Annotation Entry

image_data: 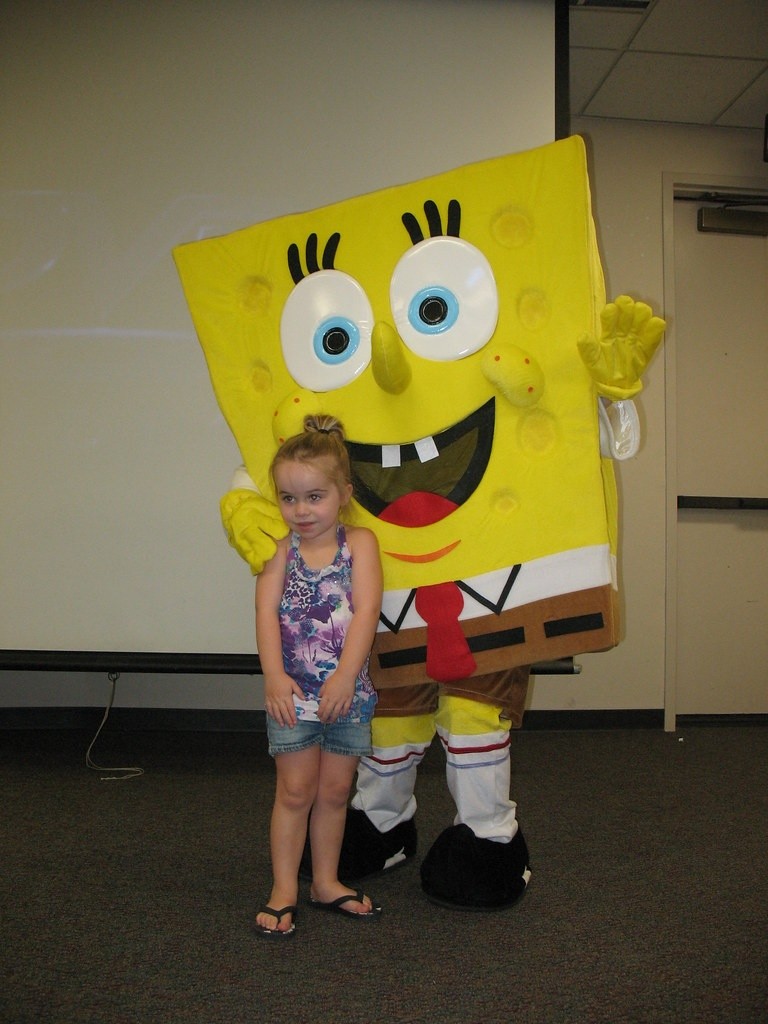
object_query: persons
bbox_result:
[254,415,384,941]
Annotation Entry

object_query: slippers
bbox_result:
[305,887,382,917]
[251,903,299,938]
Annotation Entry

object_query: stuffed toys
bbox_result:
[173,134,667,914]
